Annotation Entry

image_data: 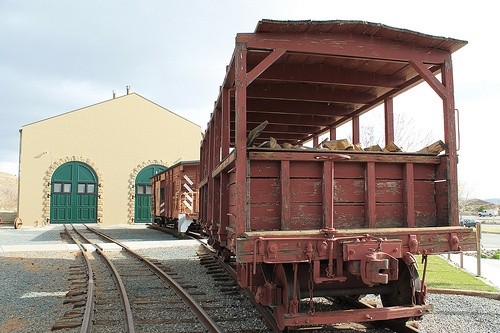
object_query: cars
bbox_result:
[479,210,498,217]
[461,218,480,228]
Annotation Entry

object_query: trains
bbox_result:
[196,17,482,333]
[148,159,202,239]
